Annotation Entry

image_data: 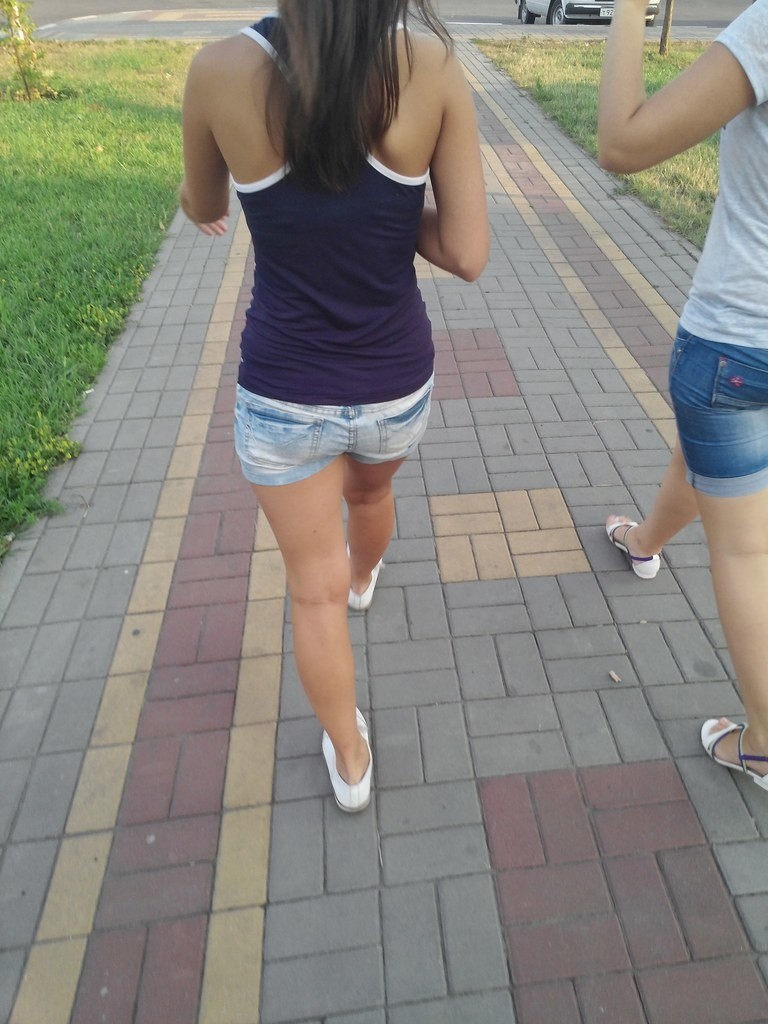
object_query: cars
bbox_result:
[515,0,661,25]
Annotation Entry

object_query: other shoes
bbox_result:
[322,707,373,814]
[347,543,382,610]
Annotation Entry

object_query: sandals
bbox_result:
[701,719,768,790]
[606,518,661,579]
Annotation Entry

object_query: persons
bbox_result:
[593,0,768,786]
[180,0,490,814]
